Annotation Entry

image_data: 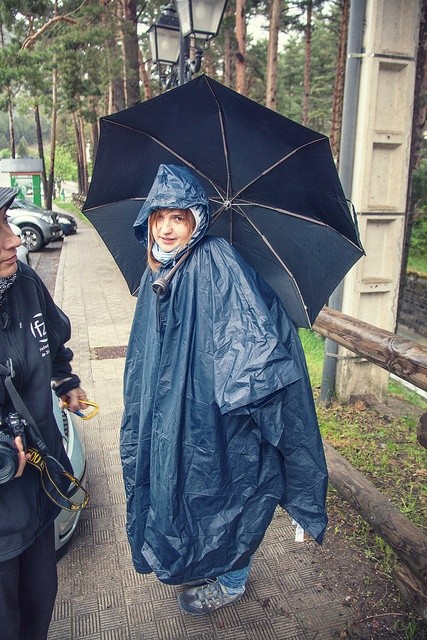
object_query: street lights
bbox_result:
[145,0,230,88]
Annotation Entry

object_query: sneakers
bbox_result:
[178,577,246,616]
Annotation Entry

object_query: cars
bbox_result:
[6,222,31,269]
[49,210,79,236]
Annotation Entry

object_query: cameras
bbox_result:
[0,412,29,485]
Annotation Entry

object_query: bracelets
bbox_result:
[51,374,81,396]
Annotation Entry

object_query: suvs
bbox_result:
[5,191,64,253]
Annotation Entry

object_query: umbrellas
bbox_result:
[82,73,366,331]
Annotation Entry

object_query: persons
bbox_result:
[119,164,328,615]
[0,187,88,640]
